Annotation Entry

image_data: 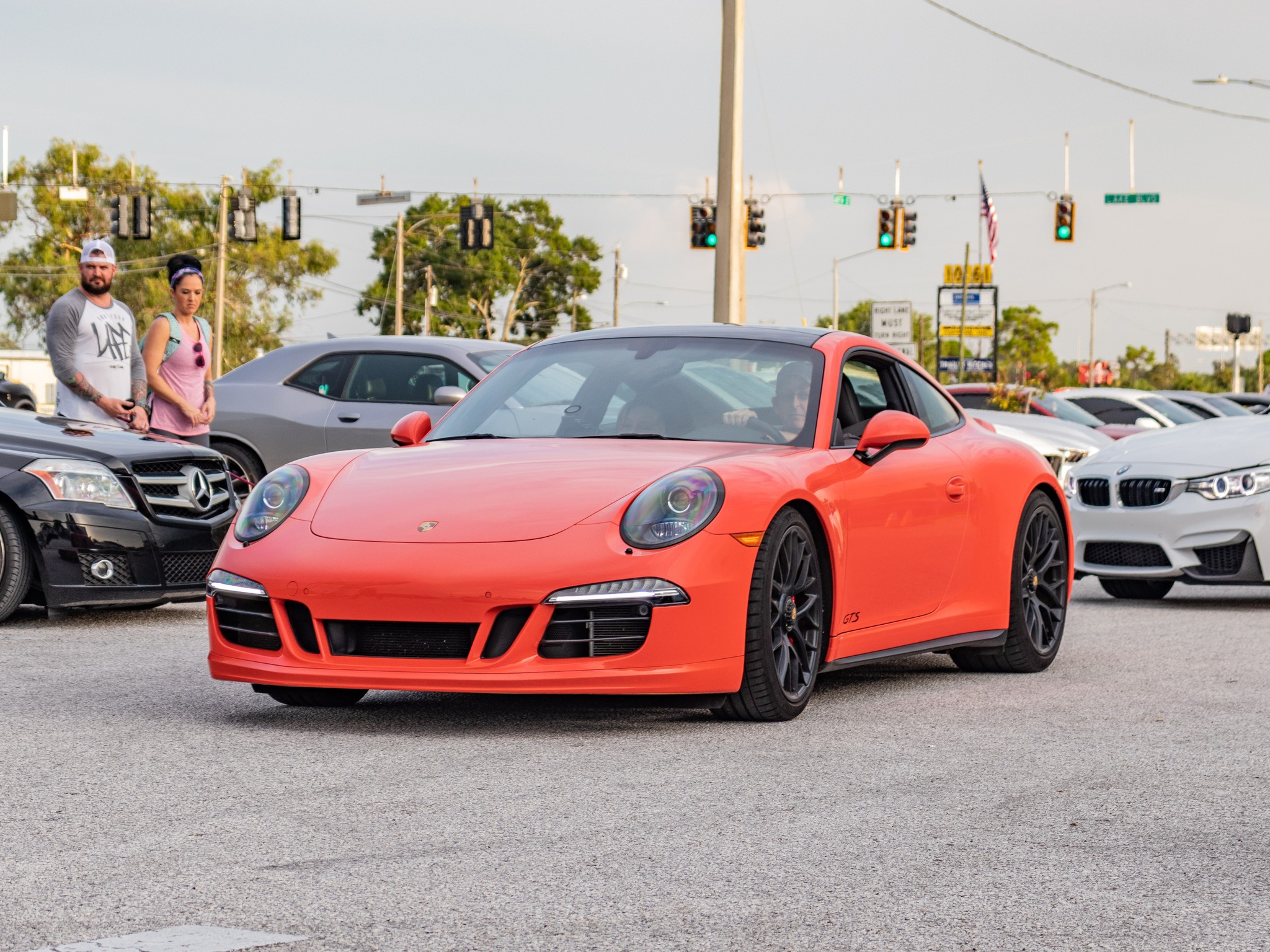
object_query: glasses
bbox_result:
[192,342,205,368]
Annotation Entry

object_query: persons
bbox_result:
[139,252,217,448]
[44,239,153,433]
[720,361,840,452]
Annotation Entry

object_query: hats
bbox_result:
[80,239,116,266]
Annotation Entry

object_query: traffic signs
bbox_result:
[1104,194,1160,203]
[834,193,850,205]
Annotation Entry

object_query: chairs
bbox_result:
[414,374,443,403]
[836,374,863,437]
[358,371,387,402]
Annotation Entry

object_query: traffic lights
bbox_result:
[1228,315,1250,337]
[878,207,897,249]
[691,205,717,249]
[227,196,257,242]
[744,202,766,250]
[1054,201,1075,243]
[110,194,127,239]
[901,208,918,250]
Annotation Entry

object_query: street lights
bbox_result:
[1089,282,1133,386]
[394,210,523,334]
[613,299,669,327]
[832,242,901,331]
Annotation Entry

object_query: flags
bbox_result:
[979,175,1001,266]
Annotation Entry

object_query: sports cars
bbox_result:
[207,326,1076,723]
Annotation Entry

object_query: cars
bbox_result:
[0,407,241,623]
[1061,414,1270,601]
[0,372,37,412]
[208,330,627,503]
[615,356,1270,491]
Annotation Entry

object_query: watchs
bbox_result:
[135,401,151,416]
